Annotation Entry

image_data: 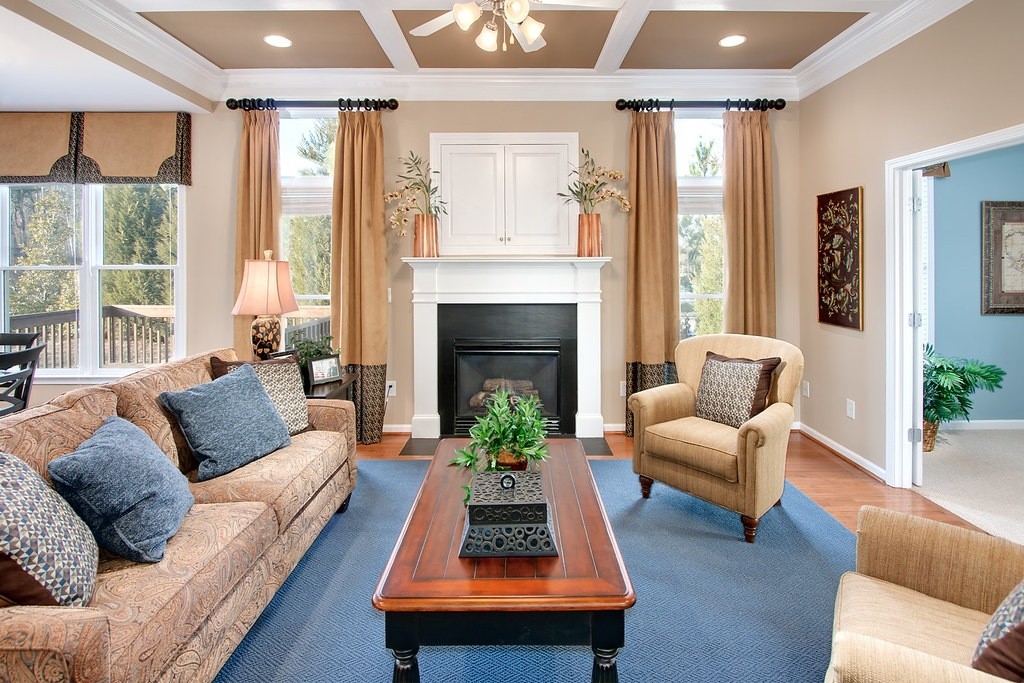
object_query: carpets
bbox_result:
[211,458,857,683]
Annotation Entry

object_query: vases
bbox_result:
[413,211,439,257]
[578,213,603,258]
[251,315,282,361]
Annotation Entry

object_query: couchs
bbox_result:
[628,333,803,542]
[0,347,357,680]
[822,506,1024,683]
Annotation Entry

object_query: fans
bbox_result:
[409,0,627,52]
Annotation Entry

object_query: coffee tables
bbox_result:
[374,437,637,682]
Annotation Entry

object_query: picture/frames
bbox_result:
[980,200,1024,314]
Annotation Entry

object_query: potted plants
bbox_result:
[922,344,1007,452]
[448,385,551,505]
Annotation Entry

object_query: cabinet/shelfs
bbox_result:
[429,132,578,256]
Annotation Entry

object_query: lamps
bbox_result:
[230,249,298,316]
[454,1,545,51]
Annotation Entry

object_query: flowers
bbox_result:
[556,147,632,214]
[382,150,449,236]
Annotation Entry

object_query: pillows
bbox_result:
[0,450,99,610]
[694,351,781,429]
[971,575,1024,683]
[160,364,292,481]
[47,415,195,564]
[211,355,317,438]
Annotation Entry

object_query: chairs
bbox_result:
[0,332,46,417]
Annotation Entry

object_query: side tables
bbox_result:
[303,373,359,401]
